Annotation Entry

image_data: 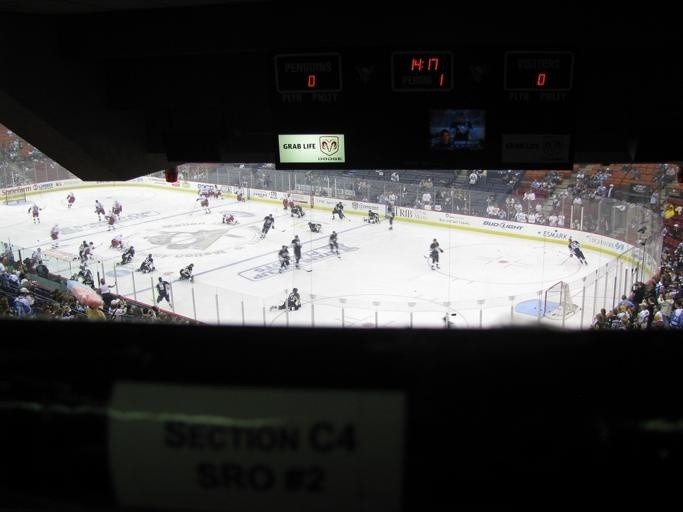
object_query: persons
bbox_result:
[196,193,211,215]
[220,214,237,224]
[0,192,195,327]
[307,222,321,232]
[289,206,304,218]
[590,165,682,329]
[282,193,294,210]
[276,245,289,274]
[566,238,588,267]
[353,162,612,233]
[329,231,341,255]
[290,235,302,267]
[429,238,443,270]
[430,110,480,147]
[269,288,301,312]
[260,214,274,237]
[331,202,343,220]
[0,125,72,191]
[181,161,353,202]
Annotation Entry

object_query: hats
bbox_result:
[19,287,29,293]
[110,298,120,305]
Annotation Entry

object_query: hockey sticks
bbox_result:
[343,216,351,221]
[289,258,312,272]
